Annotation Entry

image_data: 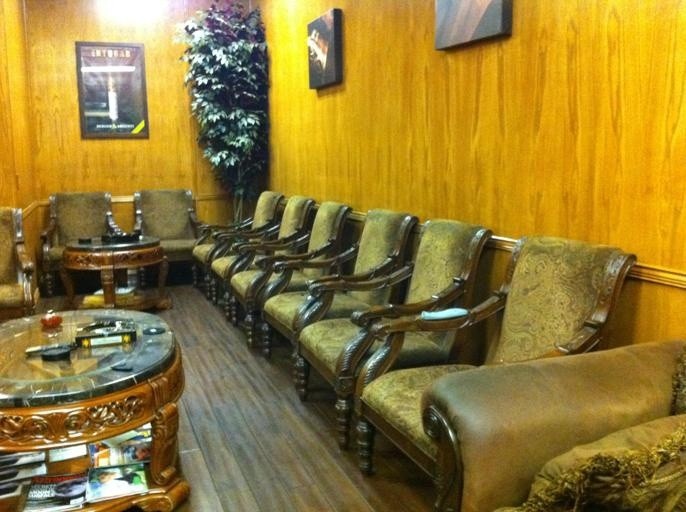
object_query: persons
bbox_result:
[307,18,336,88]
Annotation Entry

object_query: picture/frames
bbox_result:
[73,38,152,143]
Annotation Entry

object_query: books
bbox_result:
[0,421,154,511]
[75,329,138,347]
